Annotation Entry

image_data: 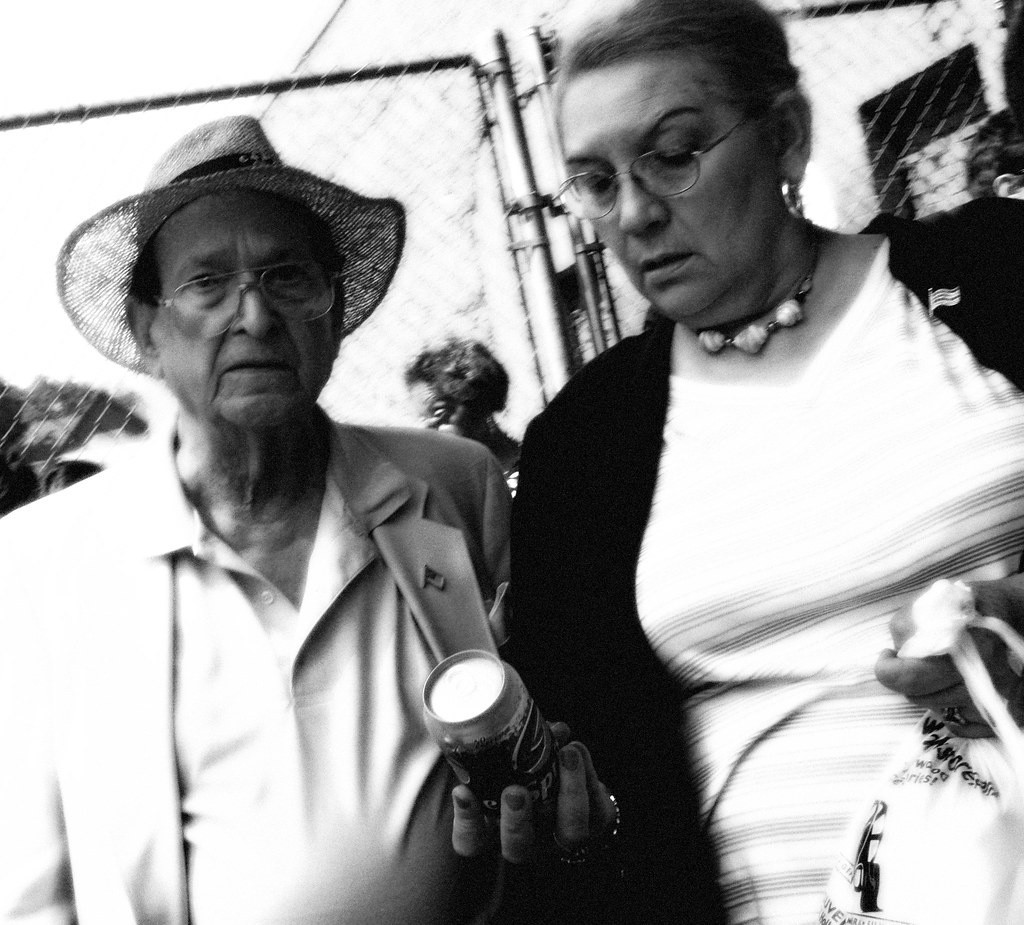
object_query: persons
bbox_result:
[965,106,1023,197]
[0,117,511,925]
[402,335,522,499]
[450,0,1023,925]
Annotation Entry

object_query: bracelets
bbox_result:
[605,795,619,836]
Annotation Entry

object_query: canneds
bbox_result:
[423,648,561,828]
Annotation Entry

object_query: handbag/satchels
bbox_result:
[820,582,1024,925]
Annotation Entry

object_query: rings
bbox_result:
[552,831,585,866]
[942,706,969,728]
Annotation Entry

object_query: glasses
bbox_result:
[551,115,747,220]
[154,261,335,337]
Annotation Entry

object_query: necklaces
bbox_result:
[698,218,819,356]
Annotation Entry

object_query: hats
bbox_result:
[57,115,407,378]
